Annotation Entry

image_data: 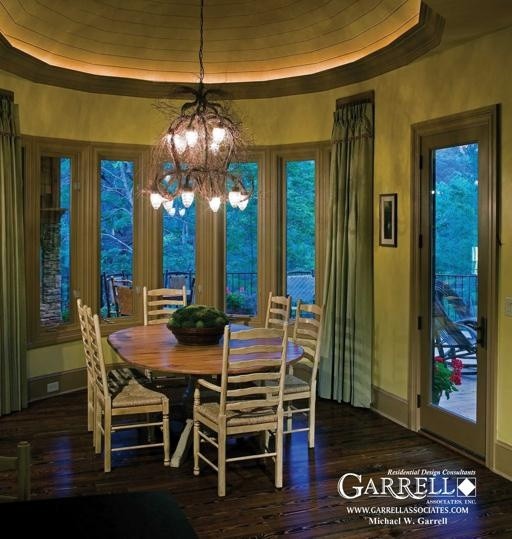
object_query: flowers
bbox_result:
[432,357,464,406]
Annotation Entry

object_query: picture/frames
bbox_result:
[379,193,397,247]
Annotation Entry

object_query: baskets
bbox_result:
[166,323,226,347]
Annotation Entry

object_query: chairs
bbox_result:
[432,279,478,377]
[0,440,33,500]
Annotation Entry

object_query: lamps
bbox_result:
[141,0,254,218]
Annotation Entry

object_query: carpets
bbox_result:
[0,489,198,539]
